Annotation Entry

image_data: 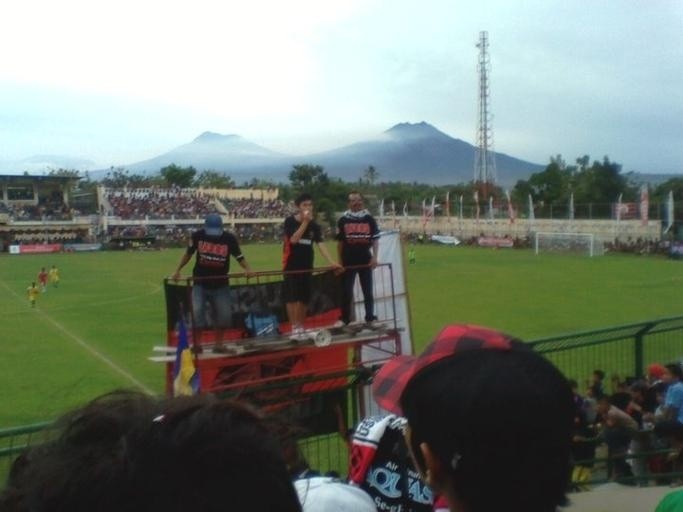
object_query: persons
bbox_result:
[48,265,59,288]
[25,282,38,308]
[566,364,682,489]
[370,323,577,512]
[418,231,533,250]
[0,387,302,512]
[37,267,47,293]
[334,191,379,329]
[240,294,282,338]
[1,192,298,244]
[272,402,452,512]
[279,192,345,335]
[603,235,682,261]
[171,214,252,356]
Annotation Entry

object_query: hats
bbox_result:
[372,325,575,451]
[203,214,224,236]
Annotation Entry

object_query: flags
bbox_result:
[164,265,357,442]
[380,193,515,226]
[616,182,674,234]
[169,317,201,397]
